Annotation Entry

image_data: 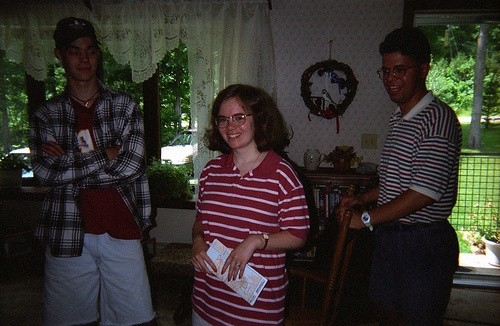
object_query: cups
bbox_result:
[303,149,327,172]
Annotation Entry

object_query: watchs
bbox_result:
[360,210,374,231]
[262,234,269,250]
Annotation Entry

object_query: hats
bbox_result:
[52,17,102,51]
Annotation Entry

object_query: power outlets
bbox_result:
[361,135,379,150]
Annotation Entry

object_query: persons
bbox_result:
[331,25,461,326]
[190,84,310,326]
[29,17,157,326]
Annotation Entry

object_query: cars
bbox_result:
[162,129,198,178]
[0,147,34,178]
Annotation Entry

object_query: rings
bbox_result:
[237,263,241,267]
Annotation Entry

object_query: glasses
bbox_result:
[215,114,254,127]
[376,65,419,80]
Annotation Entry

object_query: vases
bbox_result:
[331,157,352,169]
[482,231,500,265]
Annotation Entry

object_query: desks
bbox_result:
[301,171,382,217]
[0,182,162,256]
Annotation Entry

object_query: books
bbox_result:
[308,186,343,231]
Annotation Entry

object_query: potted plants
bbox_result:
[0,152,30,187]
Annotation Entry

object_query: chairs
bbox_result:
[284,182,361,326]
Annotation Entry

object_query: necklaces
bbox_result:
[249,148,263,168]
[70,89,100,107]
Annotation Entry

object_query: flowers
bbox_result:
[463,203,500,244]
[326,145,357,165]
[301,61,358,121]
